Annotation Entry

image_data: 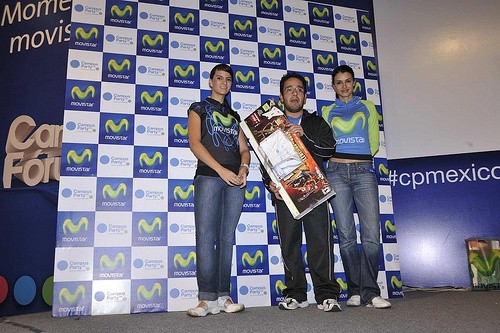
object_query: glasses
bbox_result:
[283,88,304,95]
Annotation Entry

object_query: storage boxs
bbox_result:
[238,97,335,220]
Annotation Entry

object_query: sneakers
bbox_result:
[365,297,392,307]
[346,295,360,305]
[317,299,342,312]
[279,297,309,309]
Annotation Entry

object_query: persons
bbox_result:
[321,65,392,309]
[187,63,250,317]
[259,72,342,312]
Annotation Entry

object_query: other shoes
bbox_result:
[218,296,244,313]
[187,301,220,317]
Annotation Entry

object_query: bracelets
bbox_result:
[240,163,249,169]
